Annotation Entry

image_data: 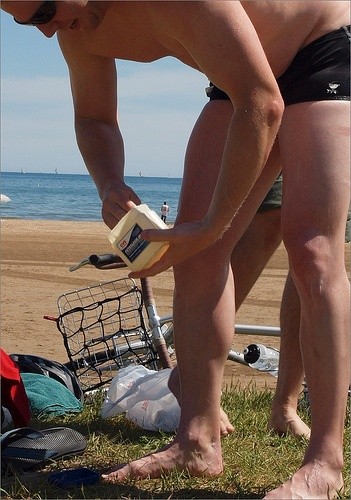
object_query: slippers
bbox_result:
[1,427,87,476]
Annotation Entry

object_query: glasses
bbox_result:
[14,0,56,27]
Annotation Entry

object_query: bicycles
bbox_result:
[55,199,282,397]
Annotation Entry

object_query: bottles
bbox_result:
[243,343,280,373]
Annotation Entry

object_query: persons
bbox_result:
[0,0,351,500]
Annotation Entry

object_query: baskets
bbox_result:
[56,278,158,396]
